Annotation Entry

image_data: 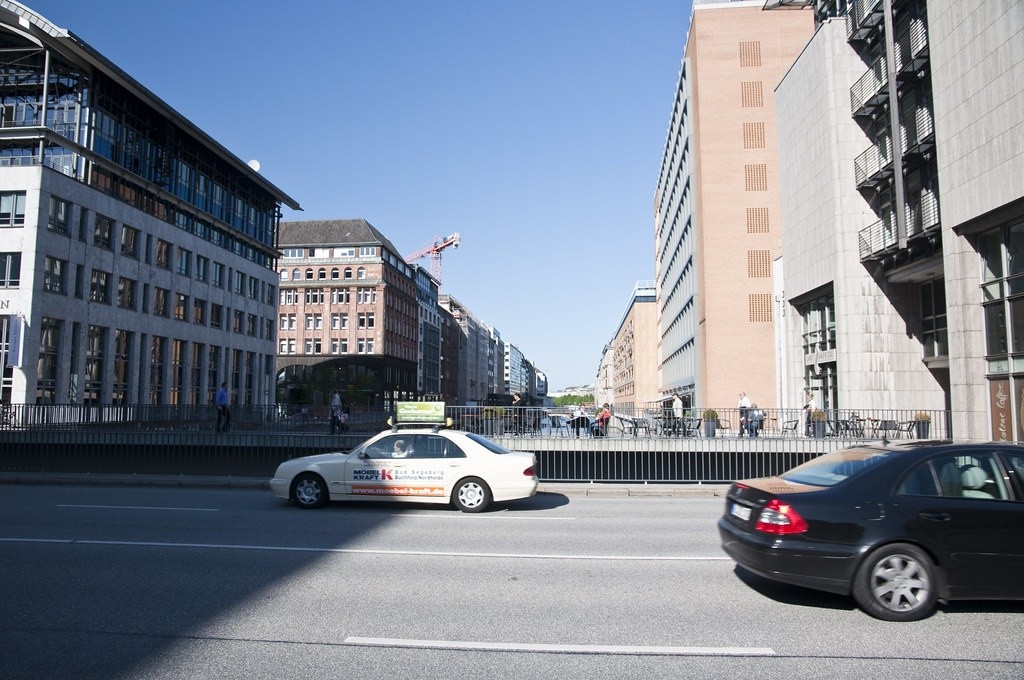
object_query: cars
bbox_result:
[271,418,539,513]
[717,438,1024,622]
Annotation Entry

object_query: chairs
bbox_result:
[654,416,680,437]
[716,419,734,438]
[631,418,651,439]
[760,418,799,438]
[526,417,542,438]
[405,442,426,457]
[547,413,571,438]
[614,415,636,438]
[827,419,866,439]
[959,464,995,499]
[682,418,702,438]
[868,418,916,440]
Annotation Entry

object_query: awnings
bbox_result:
[648,390,689,402]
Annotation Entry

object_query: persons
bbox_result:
[738,391,767,437]
[328,388,350,435]
[512,392,524,435]
[215,382,231,433]
[302,404,308,423]
[668,393,686,436]
[588,403,611,437]
[805,394,816,438]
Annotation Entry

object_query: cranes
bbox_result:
[405,232,461,294]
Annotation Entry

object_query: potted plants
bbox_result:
[914,412,931,439]
[809,407,827,438]
[481,406,506,438]
[702,409,718,437]
